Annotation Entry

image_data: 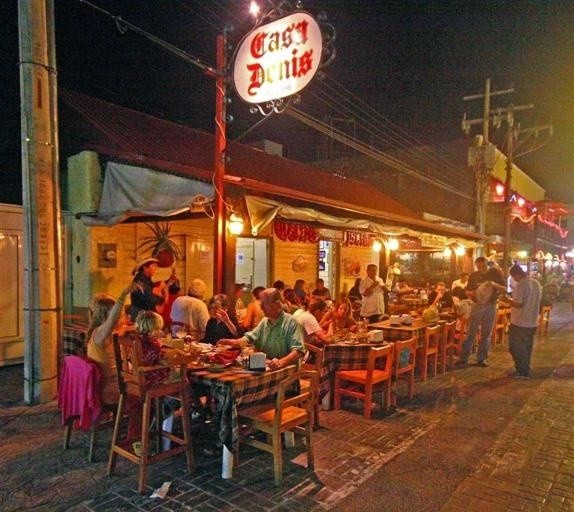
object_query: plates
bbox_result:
[209,365,225,372]
[184,362,212,369]
[194,341,214,352]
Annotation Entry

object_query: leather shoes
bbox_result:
[453,359,467,365]
[478,360,489,367]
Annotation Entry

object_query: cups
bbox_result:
[185,335,191,352]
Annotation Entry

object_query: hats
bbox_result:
[132,254,160,275]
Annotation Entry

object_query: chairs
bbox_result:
[300,299,551,429]
[63,355,115,464]
[233,370,321,486]
[107,333,195,494]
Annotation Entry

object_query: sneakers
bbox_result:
[508,371,529,379]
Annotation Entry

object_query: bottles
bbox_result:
[362,317,368,334]
[358,315,362,336]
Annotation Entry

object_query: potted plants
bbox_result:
[133,222,181,267]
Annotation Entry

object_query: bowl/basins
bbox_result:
[356,334,369,343]
[172,339,184,346]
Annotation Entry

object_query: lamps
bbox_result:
[229,213,243,234]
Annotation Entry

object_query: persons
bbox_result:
[500,265,541,378]
[87,258,305,453]
[507,275,514,296]
[294,264,473,364]
[487,261,497,271]
[456,257,506,367]
